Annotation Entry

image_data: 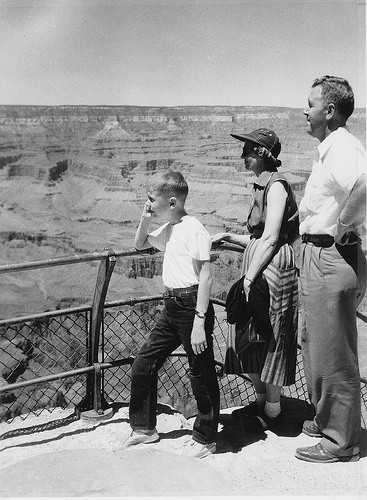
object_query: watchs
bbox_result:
[195,311,206,318]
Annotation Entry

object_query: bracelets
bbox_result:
[245,277,253,282]
[338,218,351,228]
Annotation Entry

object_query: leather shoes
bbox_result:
[294,442,360,464]
[297,419,325,437]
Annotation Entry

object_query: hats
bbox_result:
[229,127,281,159]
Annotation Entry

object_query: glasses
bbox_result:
[242,147,255,156]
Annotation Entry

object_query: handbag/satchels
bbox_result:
[234,316,267,355]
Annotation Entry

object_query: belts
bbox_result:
[164,284,199,298]
[301,233,334,245]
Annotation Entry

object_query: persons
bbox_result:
[118,169,220,458]
[287,75,367,463]
[210,128,300,431]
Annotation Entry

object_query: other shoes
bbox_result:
[181,437,216,460]
[244,411,284,434]
[232,398,265,422]
[112,426,160,452]
